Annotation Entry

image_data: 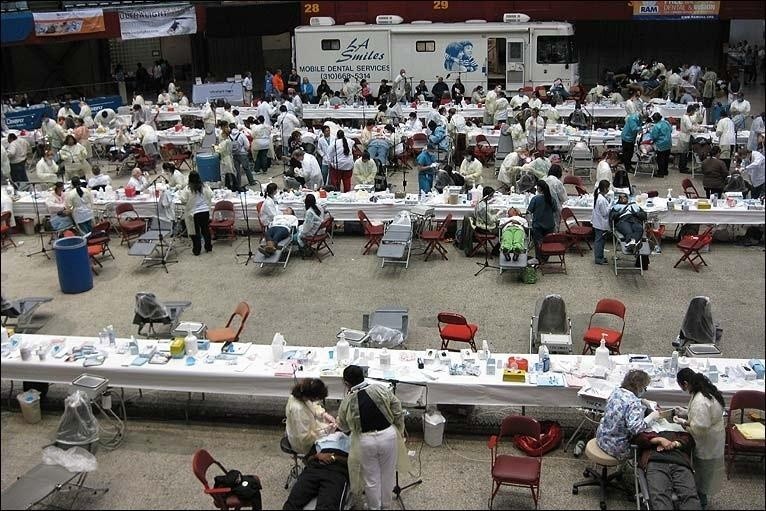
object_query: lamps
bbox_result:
[168,7,180,31]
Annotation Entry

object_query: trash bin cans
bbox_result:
[421,413,446,447]
[16,392,42,424]
[22,219,35,236]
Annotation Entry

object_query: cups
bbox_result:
[329,351,334,359]
[672,125,677,134]
[182,323,198,366]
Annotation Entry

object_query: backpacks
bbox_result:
[229,133,242,156]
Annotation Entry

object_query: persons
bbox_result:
[285,378,337,454]
[596,370,661,501]
[636,404,703,510]
[335,365,404,510]
[672,368,726,505]
[283,427,353,510]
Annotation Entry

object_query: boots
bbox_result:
[257,241,277,257]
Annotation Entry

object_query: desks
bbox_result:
[1,334,766,427]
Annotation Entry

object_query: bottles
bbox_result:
[335,332,350,364]
[533,343,550,374]
[100,185,126,200]
[663,352,720,383]
[420,183,766,214]
[545,123,620,138]
[353,348,397,368]
[212,183,404,202]
[477,341,490,362]
[1,329,45,362]
[6,182,14,197]
[147,181,167,198]
[20,129,26,136]
[573,441,586,457]
[99,326,140,355]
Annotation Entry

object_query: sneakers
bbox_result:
[654,170,669,179]
[502,247,511,261]
[625,167,635,174]
[513,247,520,261]
[680,166,691,175]
[624,239,644,253]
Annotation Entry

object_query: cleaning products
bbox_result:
[184,328,198,354]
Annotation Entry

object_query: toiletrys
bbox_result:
[666,189,673,202]
[466,180,479,202]
[272,333,286,362]
[595,333,610,365]
[336,333,349,360]
[380,346,391,366]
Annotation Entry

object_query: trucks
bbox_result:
[288,13,579,107]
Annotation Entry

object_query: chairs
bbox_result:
[630,444,650,510]
[335,309,409,349]
[608,38,766,87]
[1,82,765,276]
[1,296,54,334]
[581,298,626,355]
[2,459,108,510]
[671,296,723,358]
[437,313,479,349]
[727,390,766,479]
[133,293,192,338]
[529,295,571,355]
[205,301,249,342]
[488,416,541,510]
[192,450,261,511]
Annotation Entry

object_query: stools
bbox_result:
[573,437,633,509]
[280,437,303,489]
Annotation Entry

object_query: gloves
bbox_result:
[430,162,437,170]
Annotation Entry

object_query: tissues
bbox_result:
[438,350,452,365]
[196,340,211,350]
[424,347,435,365]
[737,363,756,380]
[460,349,474,363]
[628,353,652,362]
